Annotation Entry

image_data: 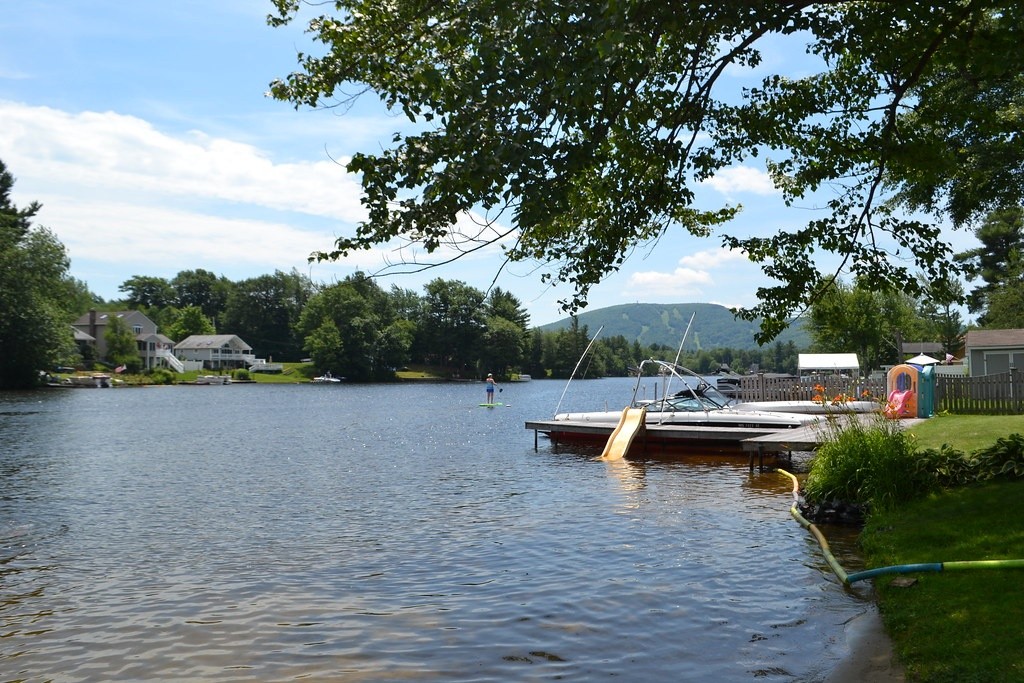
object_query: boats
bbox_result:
[510,374,532,382]
[68,371,113,389]
[538,311,883,448]
[313,372,341,384]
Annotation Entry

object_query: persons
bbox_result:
[487,373,497,403]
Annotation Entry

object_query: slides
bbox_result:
[883,389,912,417]
[599,407,646,460]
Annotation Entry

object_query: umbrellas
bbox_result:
[905,353,940,366]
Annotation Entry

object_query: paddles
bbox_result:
[495,385,504,393]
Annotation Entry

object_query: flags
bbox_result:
[115,364,126,372]
[946,353,954,363]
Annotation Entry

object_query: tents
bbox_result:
[796,352,860,382]
